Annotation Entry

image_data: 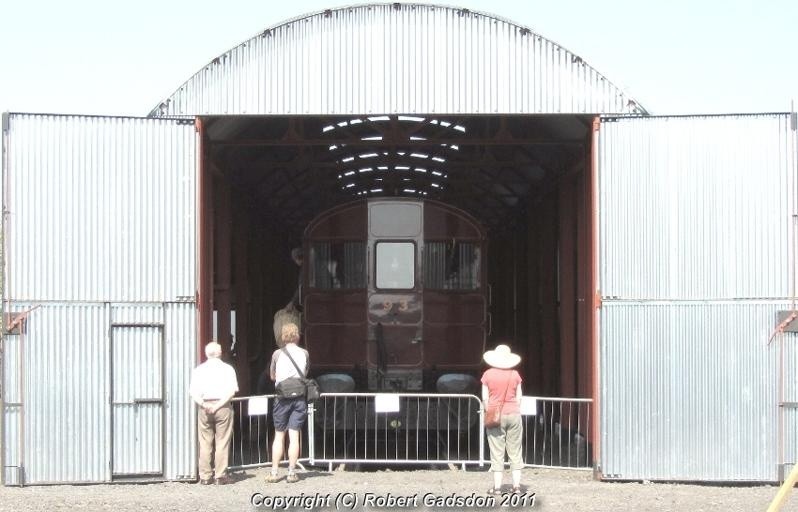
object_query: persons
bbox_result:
[481,344,523,495]
[286,247,304,312]
[190,341,239,485]
[265,323,311,483]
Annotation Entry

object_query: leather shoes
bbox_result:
[201,477,212,485]
[215,477,235,485]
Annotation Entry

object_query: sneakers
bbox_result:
[287,474,299,483]
[265,473,279,483]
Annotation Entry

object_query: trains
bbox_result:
[291,194,492,457]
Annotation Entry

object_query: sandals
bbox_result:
[487,487,503,495]
[512,487,522,494]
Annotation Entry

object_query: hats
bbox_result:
[483,345,521,369]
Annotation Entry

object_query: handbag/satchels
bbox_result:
[276,378,306,400]
[303,378,321,402]
[484,407,501,429]
